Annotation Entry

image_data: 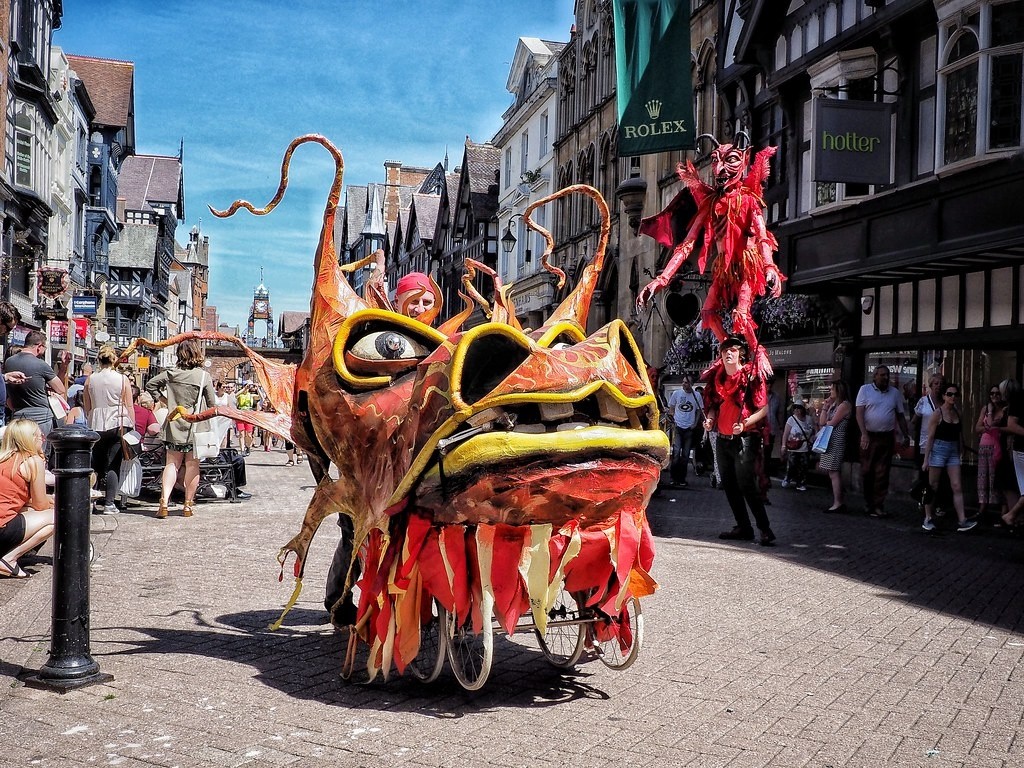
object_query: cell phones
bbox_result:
[13,376,32,383]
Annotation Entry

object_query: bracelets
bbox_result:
[822,408,828,411]
[710,418,713,420]
[741,422,745,430]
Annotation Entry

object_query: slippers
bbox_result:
[1001,518,1021,537]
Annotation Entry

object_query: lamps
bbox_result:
[500,214,531,254]
[83,233,108,267]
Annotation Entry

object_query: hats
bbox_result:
[397,272,437,298]
[790,400,806,412]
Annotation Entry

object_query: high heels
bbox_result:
[154,506,168,517]
[183,501,193,516]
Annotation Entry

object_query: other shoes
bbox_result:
[822,504,844,513]
[782,478,791,488]
[719,525,756,540]
[324,590,361,626]
[757,527,776,543]
[796,485,807,490]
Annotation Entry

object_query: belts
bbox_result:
[718,433,732,439]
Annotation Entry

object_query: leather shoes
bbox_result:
[228,489,251,500]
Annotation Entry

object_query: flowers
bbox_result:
[660,293,809,374]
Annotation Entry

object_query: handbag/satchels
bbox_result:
[193,432,219,460]
[46,391,70,419]
[121,429,142,460]
[786,440,803,449]
[812,425,834,454]
[118,456,142,498]
[908,471,935,504]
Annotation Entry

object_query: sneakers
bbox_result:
[956,517,977,531]
[922,519,936,530]
[103,504,120,514]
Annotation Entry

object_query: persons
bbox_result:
[694,386,712,472]
[324,274,435,626]
[636,143,781,382]
[0,418,54,578]
[213,379,303,468]
[668,376,705,485]
[974,386,1024,532]
[903,383,915,419]
[702,338,776,544]
[764,378,783,488]
[781,400,816,491]
[906,374,945,517]
[0,331,216,516]
[921,384,978,531]
[0,301,26,425]
[819,382,852,512]
[855,365,910,516]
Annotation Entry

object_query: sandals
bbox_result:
[297,450,303,464]
[286,459,294,467]
[0,559,34,578]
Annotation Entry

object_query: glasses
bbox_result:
[35,343,48,351]
[945,391,960,398]
[721,346,742,353]
[2,319,13,333]
[989,391,1000,396]
